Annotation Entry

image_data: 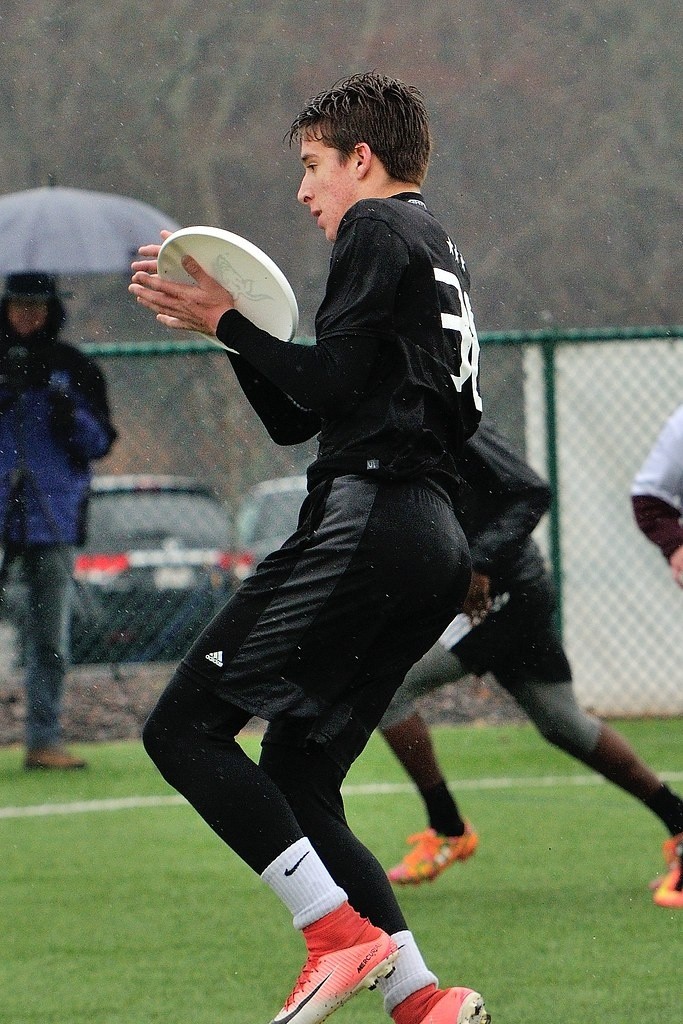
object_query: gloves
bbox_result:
[45,381,75,439]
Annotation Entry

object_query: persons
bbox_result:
[0,272,118,769]
[379,421,683,907]
[632,407,683,589]
[129,74,490,1023]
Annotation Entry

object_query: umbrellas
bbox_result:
[1,171,176,275]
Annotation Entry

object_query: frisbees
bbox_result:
[154,224,300,356]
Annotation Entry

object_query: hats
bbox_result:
[5,272,57,302]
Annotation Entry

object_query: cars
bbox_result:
[223,473,310,565]
[0,475,250,667]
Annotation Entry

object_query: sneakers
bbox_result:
[415,987,491,1024]
[28,747,85,767]
[648,834,683,906]
[387,821,478,886]
[269,926,402,1024]
